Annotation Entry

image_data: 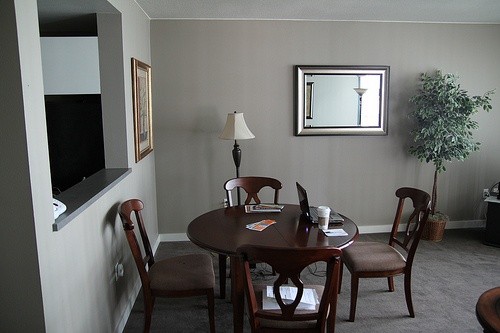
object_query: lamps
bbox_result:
[352,88,368,126]
[218,109,256,206]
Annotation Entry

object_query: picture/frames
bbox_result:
[131,57,154,163]
[306,82,315,119]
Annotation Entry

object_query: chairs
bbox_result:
[232,243,342,333]
[223,176,283,280]
[117,199,216,333]
[338,186,431,322]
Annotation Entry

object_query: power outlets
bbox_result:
[224,198,229,208]
[483,188,489,199]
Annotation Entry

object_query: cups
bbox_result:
[317,206,331,230]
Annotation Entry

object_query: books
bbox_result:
[244,203,284,213]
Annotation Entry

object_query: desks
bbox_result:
[186,203,360,333]
[475,286,500,333]
[481,195,500,248]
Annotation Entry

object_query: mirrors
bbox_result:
[295,65,391,136]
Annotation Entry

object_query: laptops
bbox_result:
[296,182,345,224]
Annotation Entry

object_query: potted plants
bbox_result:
[405,69,497,243]
